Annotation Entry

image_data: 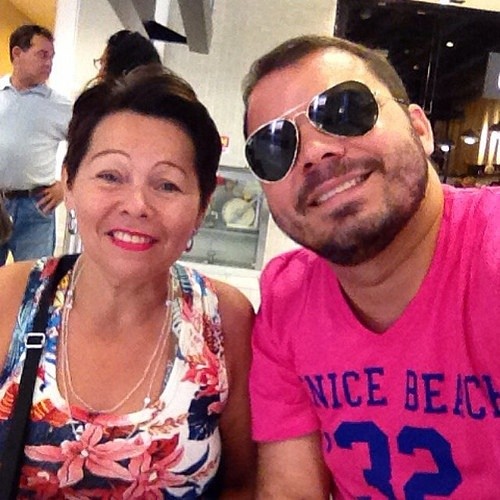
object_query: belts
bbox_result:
[0,189,43,200]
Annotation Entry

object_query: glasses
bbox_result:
[93,55,108,70]
[244,80,409,184]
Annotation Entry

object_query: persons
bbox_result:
[240,35,500,500]
[0,64,257,499]
[93,30,163,88]
[0,25,74,267]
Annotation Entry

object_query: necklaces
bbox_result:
[60,255,172,458]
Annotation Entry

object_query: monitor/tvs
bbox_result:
[334,0,492,112]
